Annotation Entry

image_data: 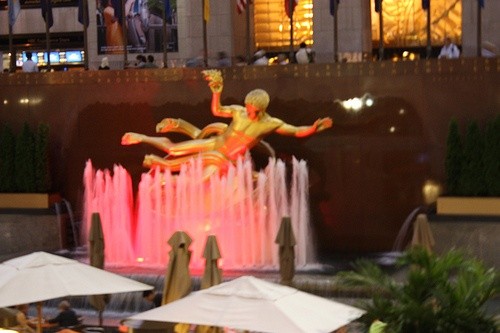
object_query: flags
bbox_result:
[236,0,250,14]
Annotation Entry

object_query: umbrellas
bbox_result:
[87,212,111,327]
[0,251,155,333]
[161,230,195,307]
[411,214,435,269]
[274,216,298,285]
[127,276,368,333]
[202,235,221,290]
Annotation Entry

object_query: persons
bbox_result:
[143,290,162,308]
[42,301,80,330]
[12,303,30,321]
[21,51,40,73]
[129,55,146,69]
[296,42,312,64]
[138,55,158,68]
[97,57,110,71]
[251,49,271,67]
[438,39,459,58]
[120,70,332,181]
[215,52,230,69]
